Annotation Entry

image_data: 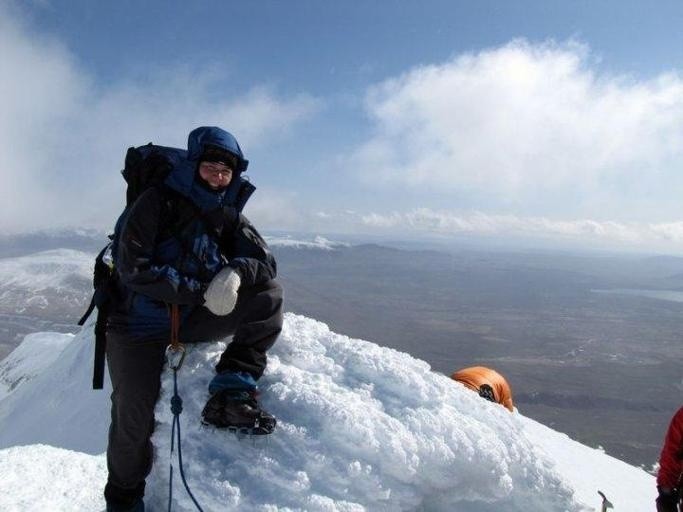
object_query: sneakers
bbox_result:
[200,388,278,434]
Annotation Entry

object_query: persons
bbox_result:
[450,363,513,411]
[78,127,286,512]
[657,407,682,512]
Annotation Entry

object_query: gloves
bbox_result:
[201,267,245,318]
